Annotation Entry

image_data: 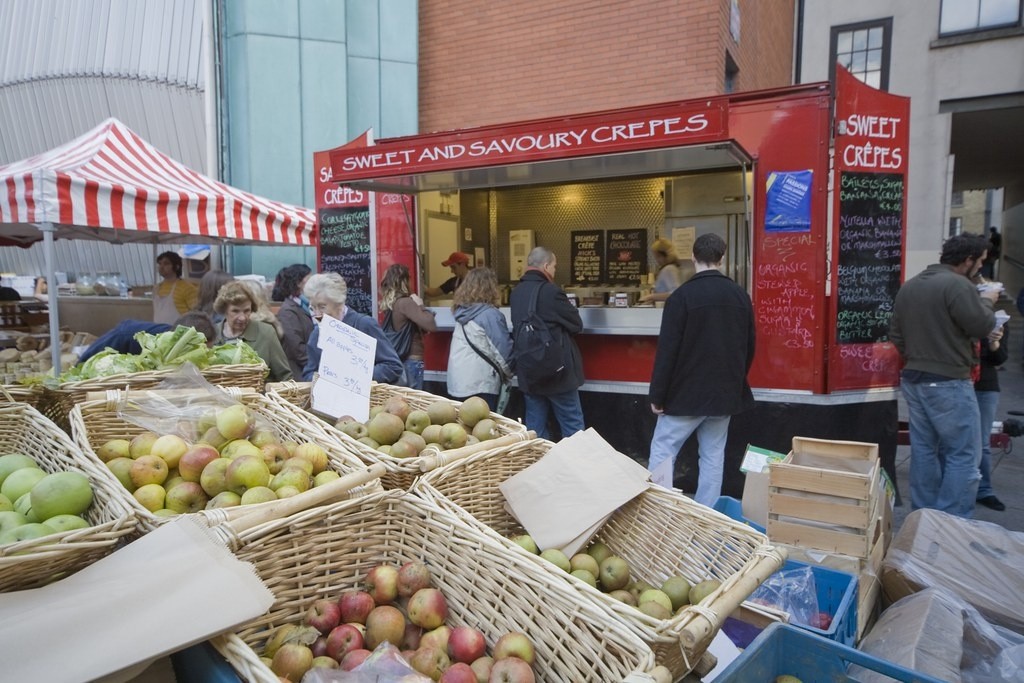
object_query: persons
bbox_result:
[272,264,318,381]
[194,270,233,323]
[74,312,215,368]
[448,266,515,413]
[301,274,403,385]
[510,248,585,440]
[379,263,437,390]
[987,226,1001,281]
[639,238,681,306]
[0,276,22,300]
[423,252,471,297]
[891,233,1009,519]
[648,232,757,507]
[213,281,294,384]
[153,251,198,325]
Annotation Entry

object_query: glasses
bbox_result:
[307,304,329,317]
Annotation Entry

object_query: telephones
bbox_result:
[571,230,604,285]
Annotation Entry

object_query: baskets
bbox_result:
[0,401,140,593]
[0,385,53,412]
[265,380,537,491]
[53,356,270,437]
[413,436,790,683]
[204,488,674,683]
[68,386,384,551]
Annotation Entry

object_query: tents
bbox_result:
[0,118,315,379]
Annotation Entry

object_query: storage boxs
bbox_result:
[710,435,1024,682]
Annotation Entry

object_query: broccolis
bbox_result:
[82,324,262,376]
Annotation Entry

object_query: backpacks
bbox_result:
[509,280,569,386]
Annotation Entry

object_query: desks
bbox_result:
[53,295,159,334]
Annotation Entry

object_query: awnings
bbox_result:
[331,138,753,259]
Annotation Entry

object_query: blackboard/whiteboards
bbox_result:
[319,208,373,320]
[606,228,648,287]
[835,170,905,343]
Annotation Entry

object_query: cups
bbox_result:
[639,272,666,309]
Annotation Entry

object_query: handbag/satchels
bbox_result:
[381,295,415,363]
[496,386,526,425]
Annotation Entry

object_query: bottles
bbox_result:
[502,284,516,305]
[972,272,986,284]
[78,272,133,299]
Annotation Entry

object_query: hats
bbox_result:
[652,238,674,253]
[442,252,469,267]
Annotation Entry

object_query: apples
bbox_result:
[509,535,720,621]
[0,453,92,545]
[334,394,501,459]
[258,564,536,683]
[95,402,340,519]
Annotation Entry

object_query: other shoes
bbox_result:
[976,496,1005,511]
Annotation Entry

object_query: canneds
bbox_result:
[566,293,576,308]
[614,293,627,308]
